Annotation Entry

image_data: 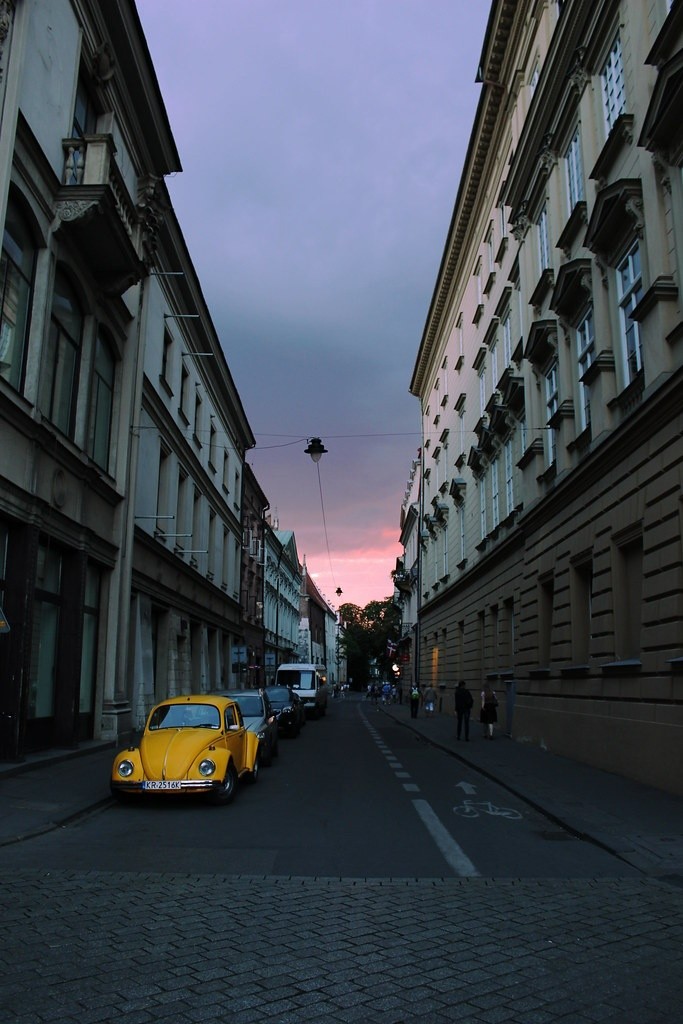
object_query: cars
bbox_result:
[109,695,261,805]
[264,685,306,739]
[206,688,279,769]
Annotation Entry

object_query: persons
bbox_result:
[288,689,305,725]
[367,680,401,705]
[452,680,475,742]
[317,678,328,717]
[423,684,438,719]
[329,680,349,701]
[409,682,420,719]
[481,684,500,741]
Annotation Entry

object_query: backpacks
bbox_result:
[411,687,419,700]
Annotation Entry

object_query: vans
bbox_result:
[275,663,329,721]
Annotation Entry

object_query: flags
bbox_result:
[386,642,399,659]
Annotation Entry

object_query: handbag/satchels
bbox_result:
[493,694,498,707]
